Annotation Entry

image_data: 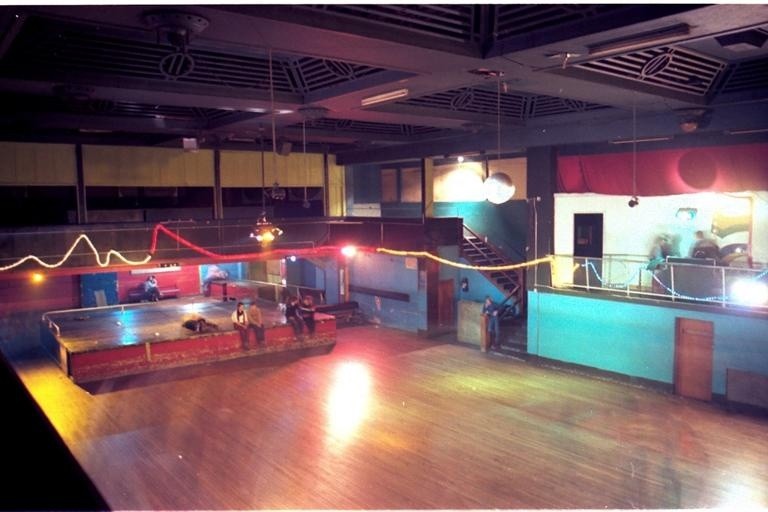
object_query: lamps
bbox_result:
[481,72,517,206]
[250,131,284,253]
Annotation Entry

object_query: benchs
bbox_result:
[128,284,181,301]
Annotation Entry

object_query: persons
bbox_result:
[231,302,252,351]
[647,229,718,272]
[285,295,305,342]
[480,296,502,345]
[247,301,267,348]
[150,276,159,302]
[299,295,318,339]
[144,275,163,298]
